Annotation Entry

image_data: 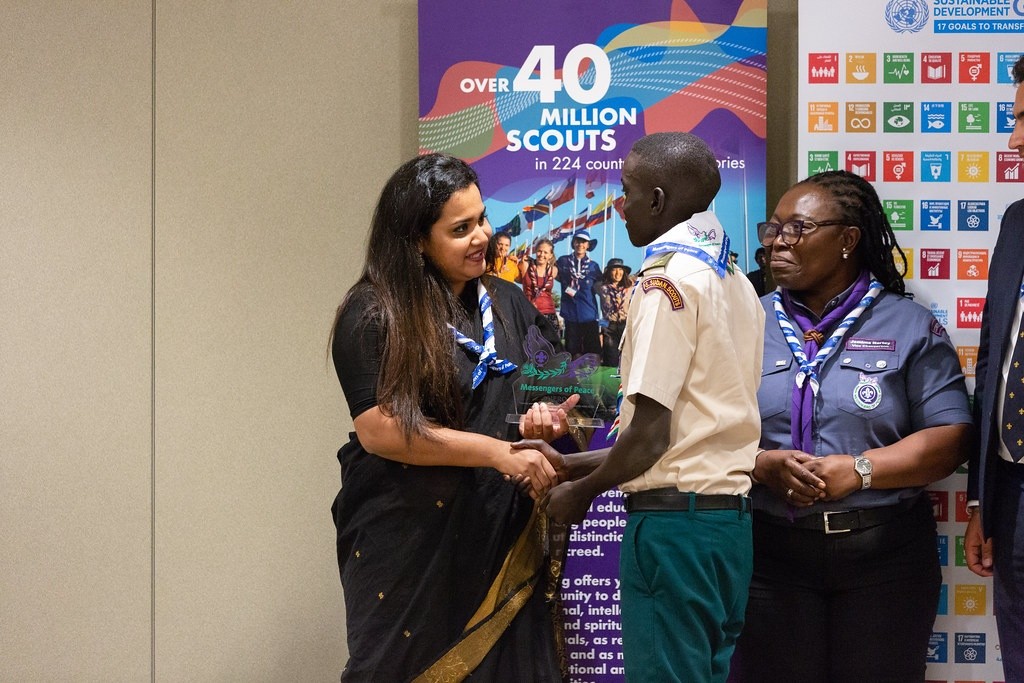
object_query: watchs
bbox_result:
[850,453,872,490]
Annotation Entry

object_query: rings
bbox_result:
[785,489,794,499]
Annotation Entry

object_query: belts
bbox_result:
[755,496,918,535]
[625,486,754,512]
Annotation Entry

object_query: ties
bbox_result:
[446,278,517,390]
[772,273,885,397]
[783,271,869,457]
[1002,311,1024,465]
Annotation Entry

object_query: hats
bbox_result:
[604,258,632,276]
[571,229,598,251]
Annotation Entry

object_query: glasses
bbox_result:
[757,220,866,247]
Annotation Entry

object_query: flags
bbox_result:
[495,172,626,259]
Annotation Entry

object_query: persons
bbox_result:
[486,231,776,366]
[503,132,767,683]
[730,169,973,683]
[962,56,1024,683]
[329,154,596,683]
